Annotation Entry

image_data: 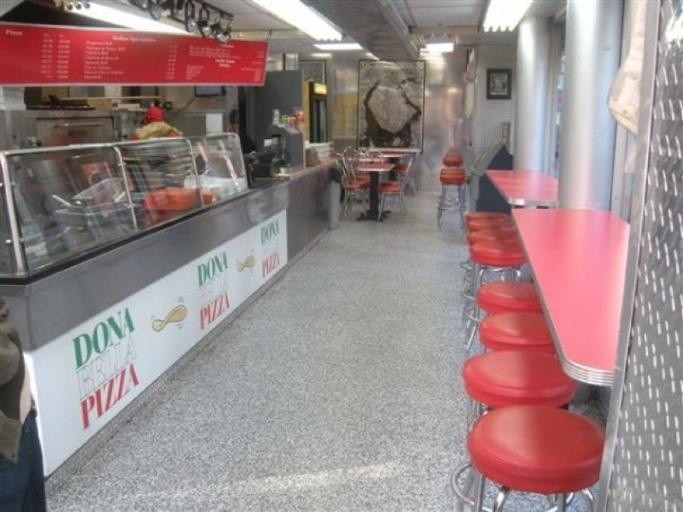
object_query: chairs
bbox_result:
[340,144,418,224]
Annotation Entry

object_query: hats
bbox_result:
[140,107,163,124]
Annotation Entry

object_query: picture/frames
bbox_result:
[487,68,511,98]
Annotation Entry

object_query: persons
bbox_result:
[131,105,183,142]
[1,298,47,511]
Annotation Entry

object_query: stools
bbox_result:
[451,312,603,512]
[437,146,466,226]
[462,210,545,313]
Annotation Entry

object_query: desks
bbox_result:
[513,208,631,386]
[485,170,560,207]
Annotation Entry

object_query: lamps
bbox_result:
[130,0,234,43]
